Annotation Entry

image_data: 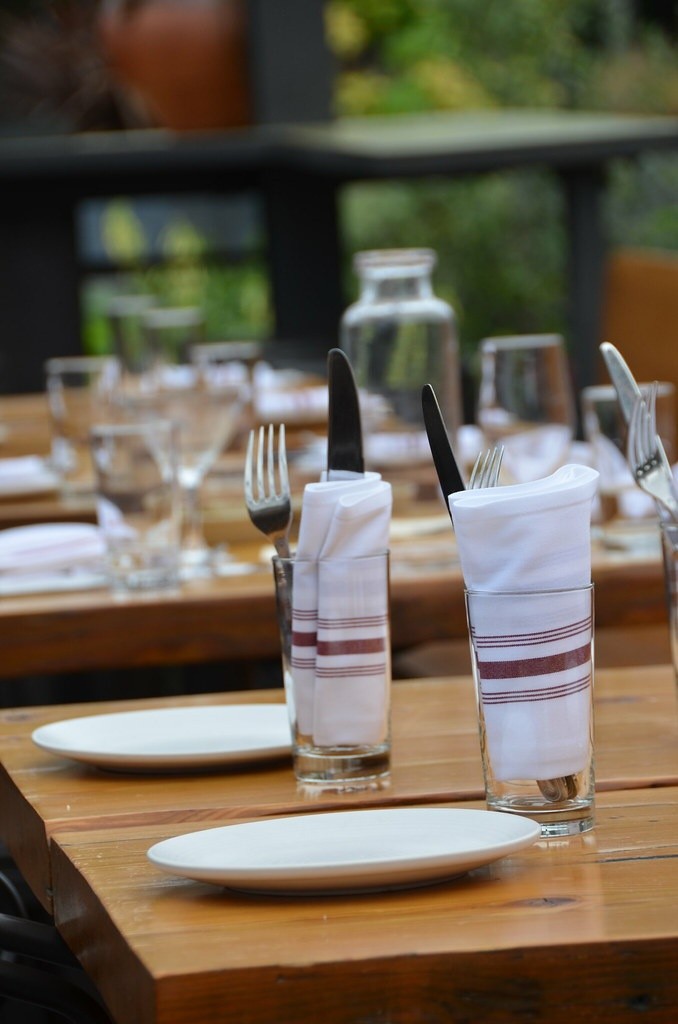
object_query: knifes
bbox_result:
[421,383,466,530]
[600,340,675,525]
[326,348,367,482]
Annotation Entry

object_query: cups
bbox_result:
[87,421,188,592]
[583,381,674,548]
[139,307,201,390]
[273,550,392,790]
[42,357,118,510]
[658,523,678,674]
[463,583,599,839]
[102,295,159,375]
[191,343,258,418]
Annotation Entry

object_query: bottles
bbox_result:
[339,249,459,537]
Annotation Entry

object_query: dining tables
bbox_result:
[0,359,678,683]
[1,664,677,1024]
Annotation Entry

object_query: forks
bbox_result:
[241,422,295,602]
[468,444,504,491]
[625,379,677,523]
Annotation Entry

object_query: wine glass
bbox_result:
[115,386,244,578]
[477,333,574,485]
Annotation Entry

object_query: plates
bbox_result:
[31,702,296,775]
[147,809,542,899]
